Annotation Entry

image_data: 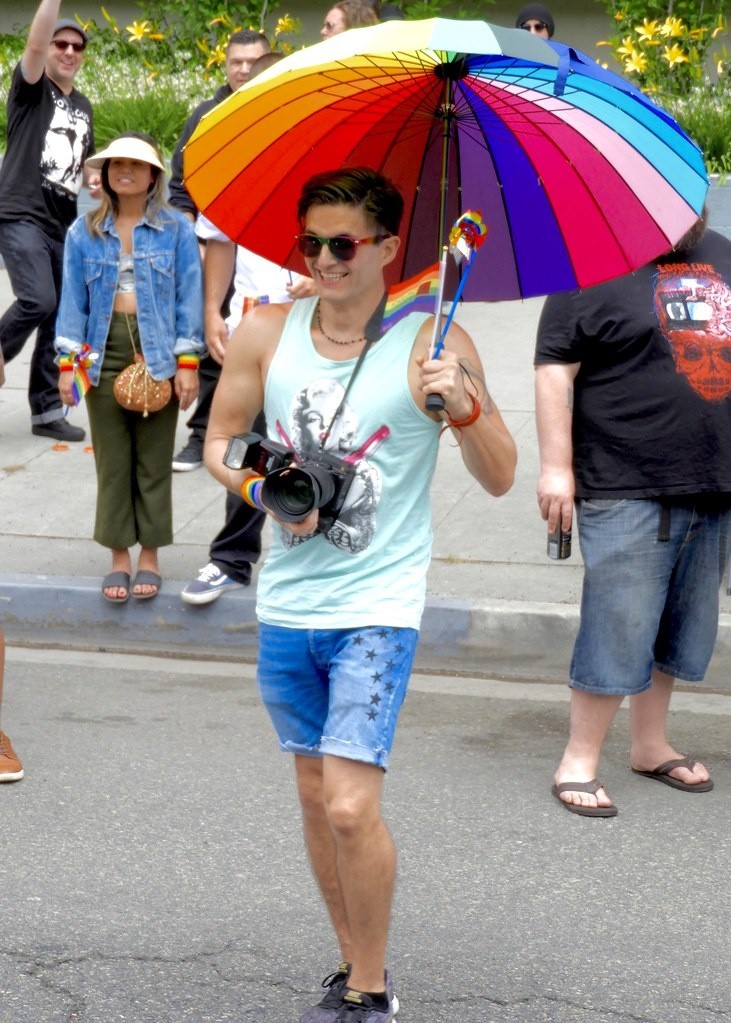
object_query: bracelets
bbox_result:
[241,475,268,513]
[177,353,199,370]
[438,392,481,447]
[59,352,73,372]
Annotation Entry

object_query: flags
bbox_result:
[71,364,92,407]
[380,261,439,331]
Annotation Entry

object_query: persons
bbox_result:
[203,165,516,1023]
[0,0,102,441]
[0,624,23,783]
[515,4,555,40]
[167,30,271,222]
[171,53,289,470]
[54,132,204,603]
[534,203,731,818]
[321,0,380,39]
[180,207,320,606]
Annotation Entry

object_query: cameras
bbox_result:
[224,432,355,533]
[546,506,571,560]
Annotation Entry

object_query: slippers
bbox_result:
[552,778,618,818]
[131,570,162,599]
[630,756,714,794]
[101,570,130,602]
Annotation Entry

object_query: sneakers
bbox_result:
[181,562,246,604]
[329,967,395,1023]
[0,731,24,782]
[300,961,400,1023]
[172,448,204,471]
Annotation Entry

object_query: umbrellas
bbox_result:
[181,17,708,411]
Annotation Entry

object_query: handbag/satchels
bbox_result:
[115,360,172,419]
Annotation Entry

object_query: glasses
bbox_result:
[50,40,86,53]
[321,21,348,31]
[518,22,546,32]
[294,225,391,263]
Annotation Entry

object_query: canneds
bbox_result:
[547,515,571,560]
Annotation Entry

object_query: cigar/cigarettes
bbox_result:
[89,185,96,189]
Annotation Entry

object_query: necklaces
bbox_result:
[317,300,366,344]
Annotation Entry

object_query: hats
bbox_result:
[87,139,168,175]
[52,17,89,42]
[515,3,555,40]
[379,5,405,20]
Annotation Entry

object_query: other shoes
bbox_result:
[31,416,85,441]
